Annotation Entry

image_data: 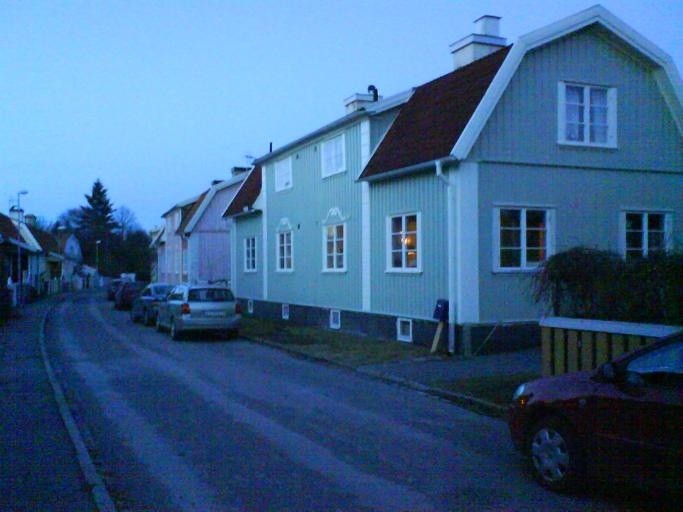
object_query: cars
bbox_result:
[509,329,683,493]
[108,278,242,340]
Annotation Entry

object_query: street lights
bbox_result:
[56,226,66,291]
[95,241,101,288]
[16,189,28,306]
